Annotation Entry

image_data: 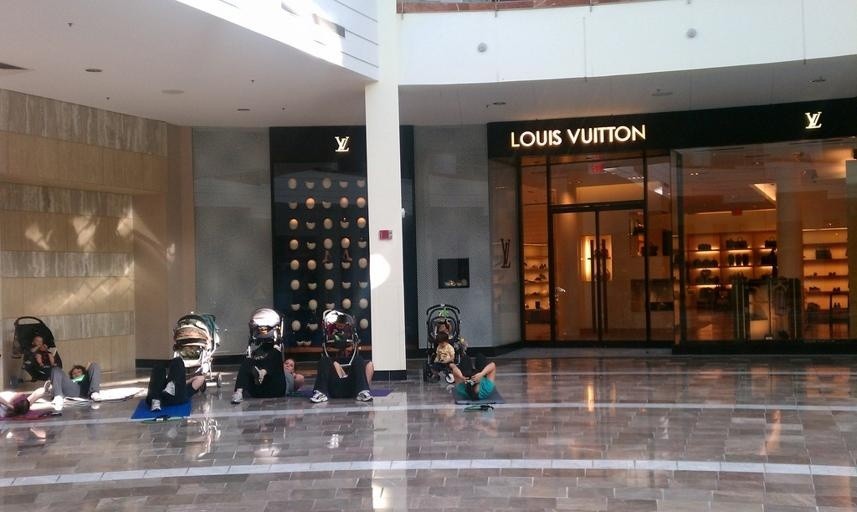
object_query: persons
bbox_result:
[145,356,205,412]
[310,402,374,454]
[0,385,46,417]
[27,336,57,367]
[236,394,307,459]
[4,427,58,456]
[429,332,456,381]
[229,346,305,405]
[143,417,191,495]
[48,360,102,405]
[325,315,353,353]
[54,405,97,441]
[435,323,453,340]
[447,352,496,401]
[308,356,375,403]
[436,399,499,448]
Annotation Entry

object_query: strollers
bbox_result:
[422,303,467,384]
[322,309,360,368]
[246,307,285,363]
[9,315,63,387]
[173,313,223,392]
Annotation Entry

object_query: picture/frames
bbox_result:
[438,258,470,288]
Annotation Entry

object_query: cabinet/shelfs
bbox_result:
[524,242,560,324]
[672,227,850,324]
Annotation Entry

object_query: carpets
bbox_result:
[452,385,505,405]
[286,389,392,397]
[34,387,148,404]
[0,408,52,420]
[130,398,192,418]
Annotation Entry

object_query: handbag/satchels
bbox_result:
[698,245,711,250]
[727,238,734,249]
[765,236,776,248]
[734,237,747,249]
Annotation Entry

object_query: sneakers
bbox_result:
[90,392,101,402]
[162,381,175,397]
[151,399,161,412]
[53,396,64,412]
[356,393,373,402]
[251,365,264,385]
[309,393,328,403]
[231,392,243,404]
